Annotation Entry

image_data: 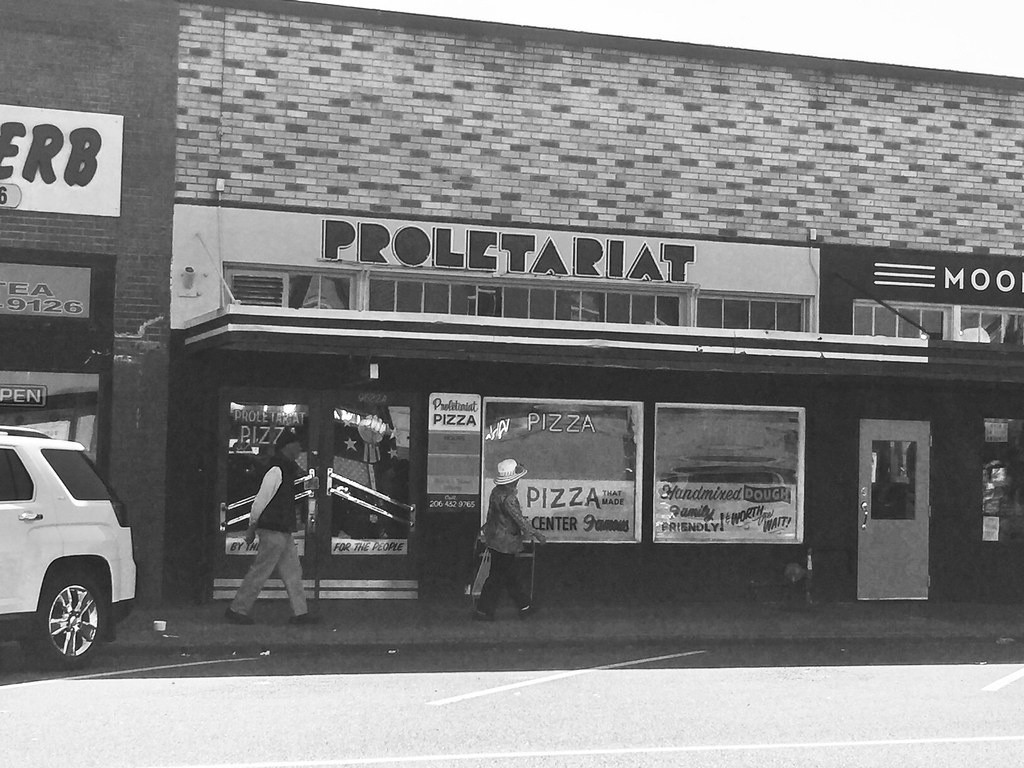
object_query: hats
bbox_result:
[493,459,528,485]
[276,432,300,450]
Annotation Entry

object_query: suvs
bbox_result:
[0,428,138,670]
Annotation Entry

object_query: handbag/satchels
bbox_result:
[472,548,492,596]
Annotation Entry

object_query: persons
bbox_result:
[469,456,550,622]
[224,432,324,625]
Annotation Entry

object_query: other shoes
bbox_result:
[290,613,319,624]
[475,610,495,621]
[519,604,540,619]
[225,607,255,625]
